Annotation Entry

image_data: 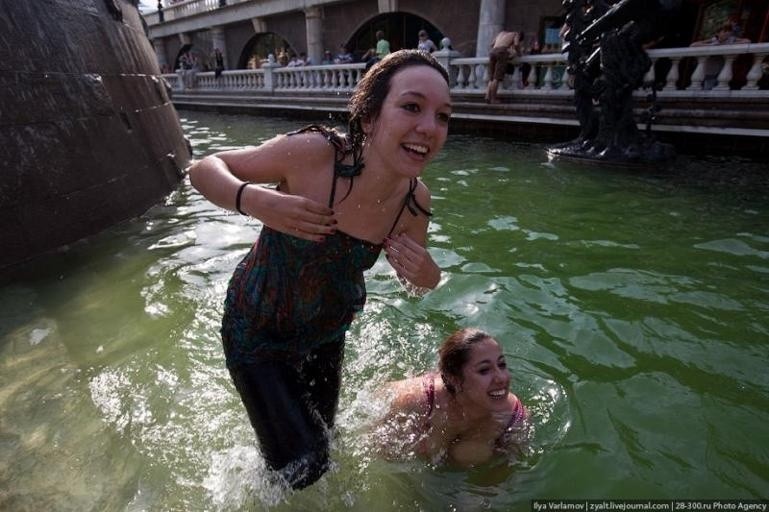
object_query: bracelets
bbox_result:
[235,182,251,218]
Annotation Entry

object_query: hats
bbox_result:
[419,29,428,40]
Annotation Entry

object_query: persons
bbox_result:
[178,54,191,69]
[378,329,529,469]
[417,29,438,54]
[189,51,454,494]
[484,25,522,104]
[375,30,390,57]
[690,27,750,89]
[249,45,354,68]
